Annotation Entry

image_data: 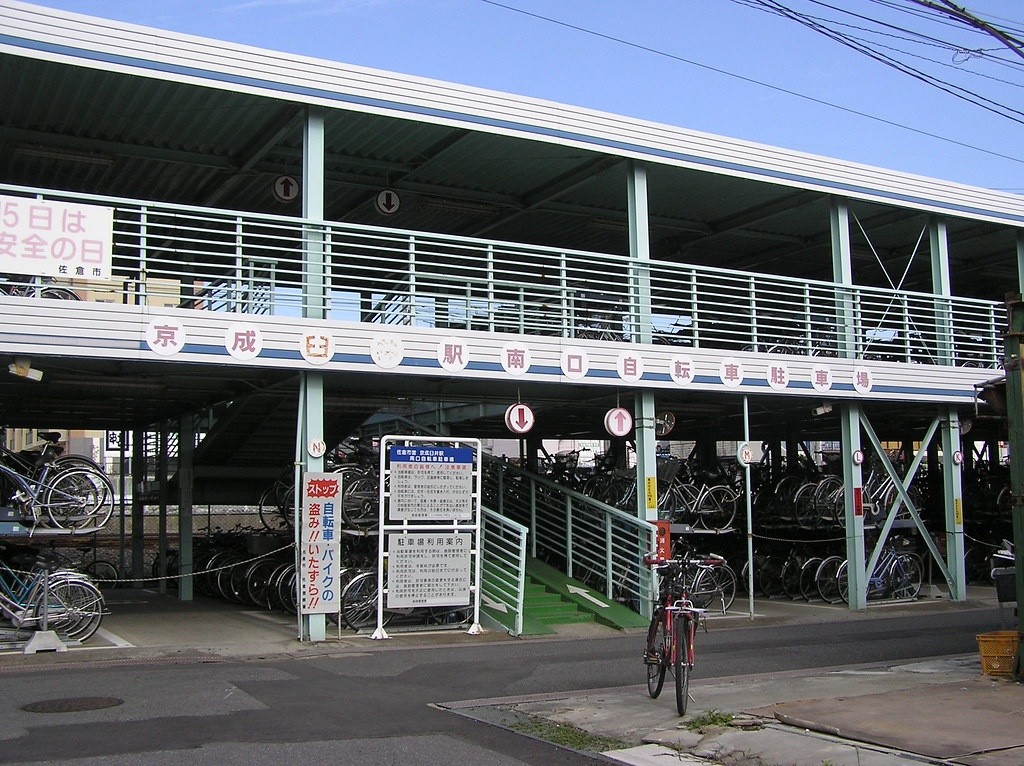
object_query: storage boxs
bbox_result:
[977,630,1020,678]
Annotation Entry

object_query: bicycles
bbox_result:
[575,303,672,345]
[642,550,725,716]
[0,272,83,302]
[0,426,1017,644]
[961,354,1005,371]
[740,323,838,358]
